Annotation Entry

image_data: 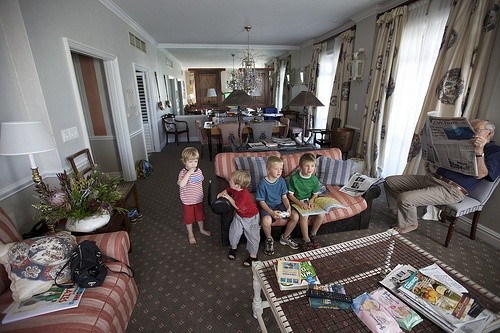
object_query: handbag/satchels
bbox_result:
[71,239,106,288]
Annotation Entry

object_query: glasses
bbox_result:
[474,127,491,133]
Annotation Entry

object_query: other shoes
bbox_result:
[135,214,143,220]
[130,218,137,224]
[310,234,320,248]
[301,239,313,252]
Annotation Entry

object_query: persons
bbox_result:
[384,119,500,234]
[217,169,260,267]
[288,153,326,251]
[255,156,299,255]
[177,147,212,244]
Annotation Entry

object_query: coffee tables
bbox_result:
[251,228,500,333]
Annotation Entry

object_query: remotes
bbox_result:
[307,289,351,302]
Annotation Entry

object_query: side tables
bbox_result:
[22,208,133,254]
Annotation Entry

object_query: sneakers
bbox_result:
[280,234,300,249]
[264,238,274,255]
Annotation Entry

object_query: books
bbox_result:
[273,256,320,290]
[292,196,347,216]
[307,286,351,308]
[2,282,85,325]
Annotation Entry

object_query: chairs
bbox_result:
[431,175,500,248]
[249,122,274,142]
[195,120,222,159]
[285,114,303,138]
[271,117,290,139]
[265,107,281,122]
[65,148,141,230]
[217,122,241,149]
[161,112,190,147]
[183,102,232,115]
[313,117,342,148]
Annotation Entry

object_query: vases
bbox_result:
[65,206,111,232]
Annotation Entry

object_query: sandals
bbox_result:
[243,256,257,267]
[228,245,239,260]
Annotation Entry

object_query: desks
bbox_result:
[263,113,284,120]
[203,120,287,162]
[229,139,317,153]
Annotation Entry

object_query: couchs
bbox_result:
[0,207,140,333]
[206,146,382,247]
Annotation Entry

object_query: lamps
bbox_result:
[0,121,64,236]
[269,64,309,88]
[352,47,366,81]
[227,26,264,96]
[220,89,257,150]
[284,90,325,148]
[206,87,217,104]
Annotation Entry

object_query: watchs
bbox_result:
[476,153,484,158]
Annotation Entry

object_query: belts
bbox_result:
[434,173,467,195]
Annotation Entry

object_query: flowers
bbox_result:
[30,164,130,233]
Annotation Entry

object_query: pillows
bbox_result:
[0,230,79,302]
[315,154,355,186]
[234,155,272,193]
